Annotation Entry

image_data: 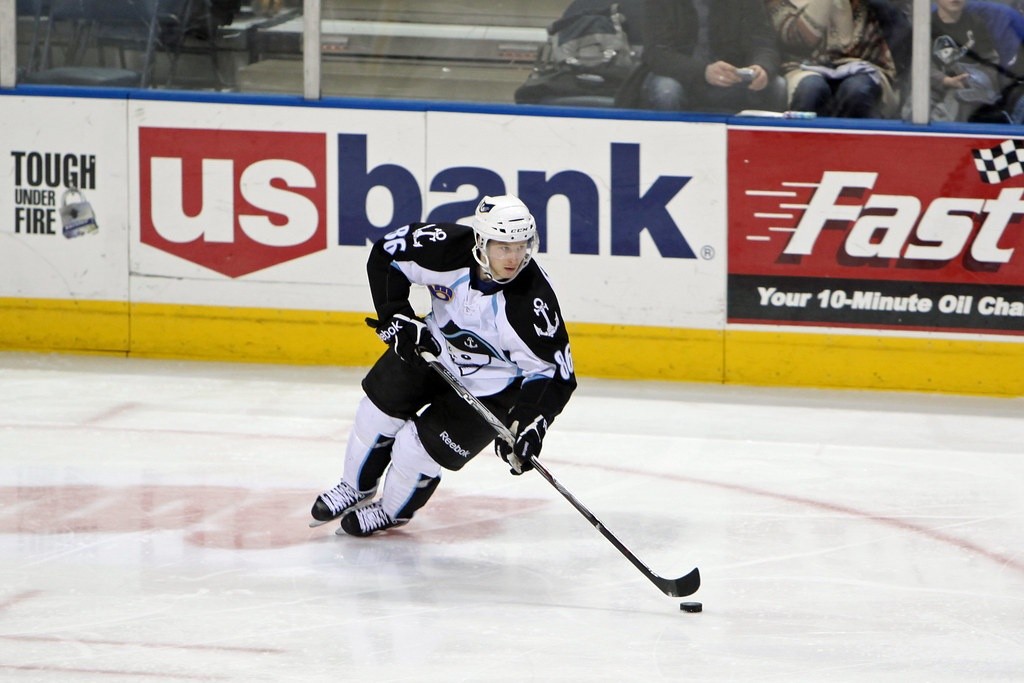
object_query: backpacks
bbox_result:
[513,0,654,106]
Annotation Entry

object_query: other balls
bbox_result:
[679,601,704,614]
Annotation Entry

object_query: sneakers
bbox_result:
[311,478,380,521]
[340,498,414,537]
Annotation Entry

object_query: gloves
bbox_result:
[494,377,568,476]
[365,300,443,372]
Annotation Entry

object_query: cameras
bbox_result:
[735,68,755,81]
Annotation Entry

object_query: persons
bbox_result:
[309,195,577,537]
[615,0,1024,124]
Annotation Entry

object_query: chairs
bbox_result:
[15,0,223,89]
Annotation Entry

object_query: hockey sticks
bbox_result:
[418,347,701,598]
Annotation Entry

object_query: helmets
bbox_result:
[470,194,539,285]
